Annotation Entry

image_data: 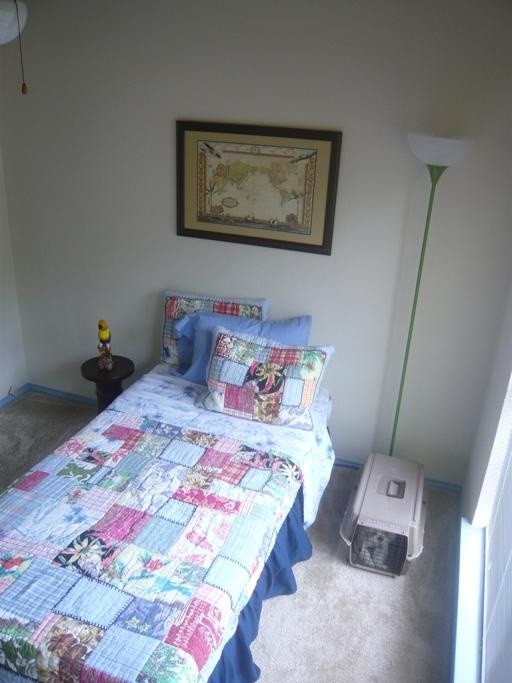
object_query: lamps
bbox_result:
[388,132,471,457]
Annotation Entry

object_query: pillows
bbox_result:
[161,291,270,364]
[174,313,312,384]
[200,326,335,431]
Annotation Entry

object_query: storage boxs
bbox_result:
[339,453,428,561]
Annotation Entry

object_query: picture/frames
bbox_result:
[175,119,342,254]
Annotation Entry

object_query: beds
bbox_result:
[0,361,336,682]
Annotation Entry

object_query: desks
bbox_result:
[81,355,134,412]
